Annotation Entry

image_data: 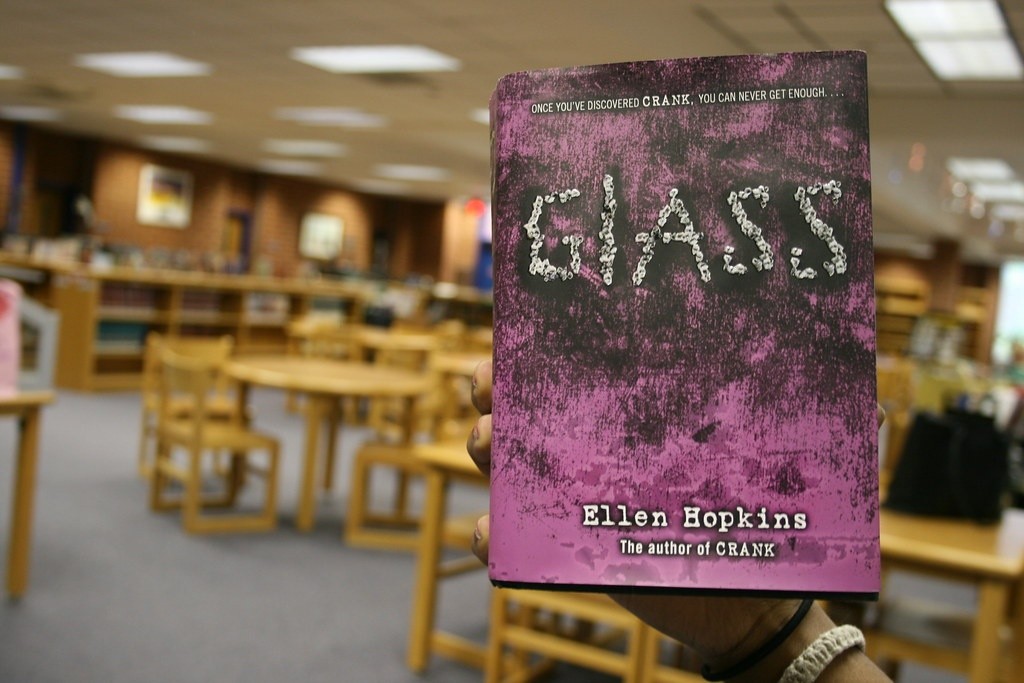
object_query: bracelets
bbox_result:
[700,596,813,683]
[780,624,866,683]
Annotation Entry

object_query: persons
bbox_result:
[467,359,893,683]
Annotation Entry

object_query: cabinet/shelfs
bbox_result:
[0,254,415,396]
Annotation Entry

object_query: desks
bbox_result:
[407,441,1024,683]
[0,390,56,602]
[222,355,428,534]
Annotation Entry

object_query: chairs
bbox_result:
[141,309,1024,683]
